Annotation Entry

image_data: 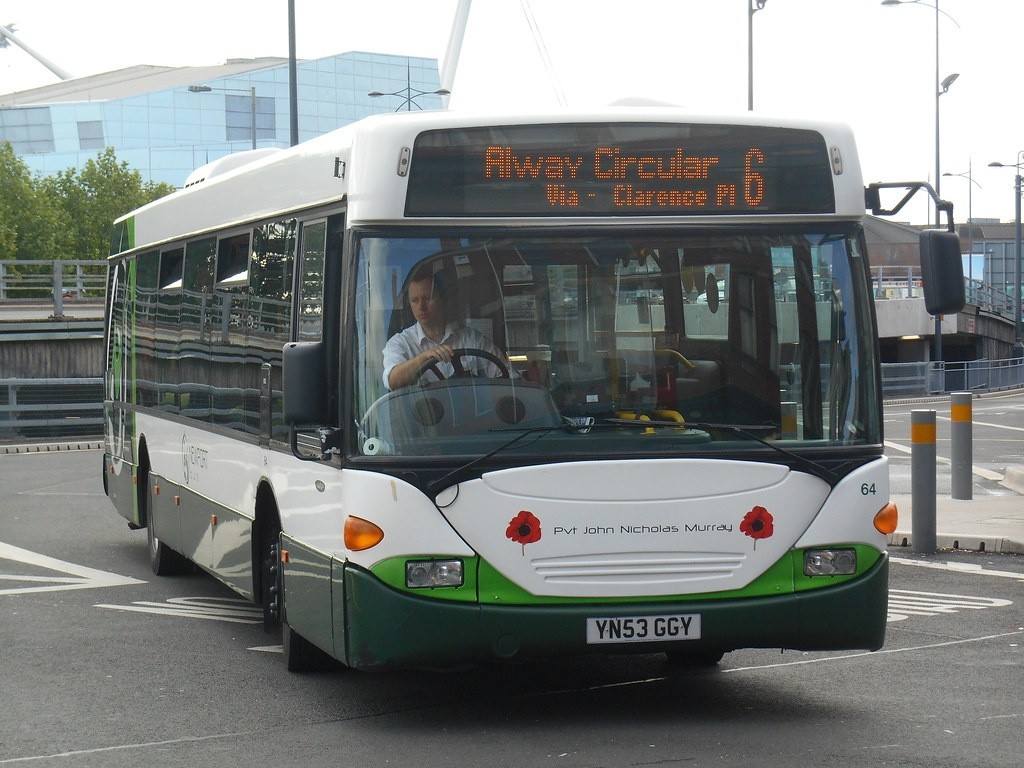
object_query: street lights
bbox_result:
[881,0,940,228]
[943,172,973,303]
[936,72,961,229]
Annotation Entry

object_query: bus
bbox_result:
[101,109,968,672]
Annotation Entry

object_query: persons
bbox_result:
[381,271,526,392]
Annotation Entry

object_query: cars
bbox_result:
[697,280,724,302]
[776,279,831,302]
